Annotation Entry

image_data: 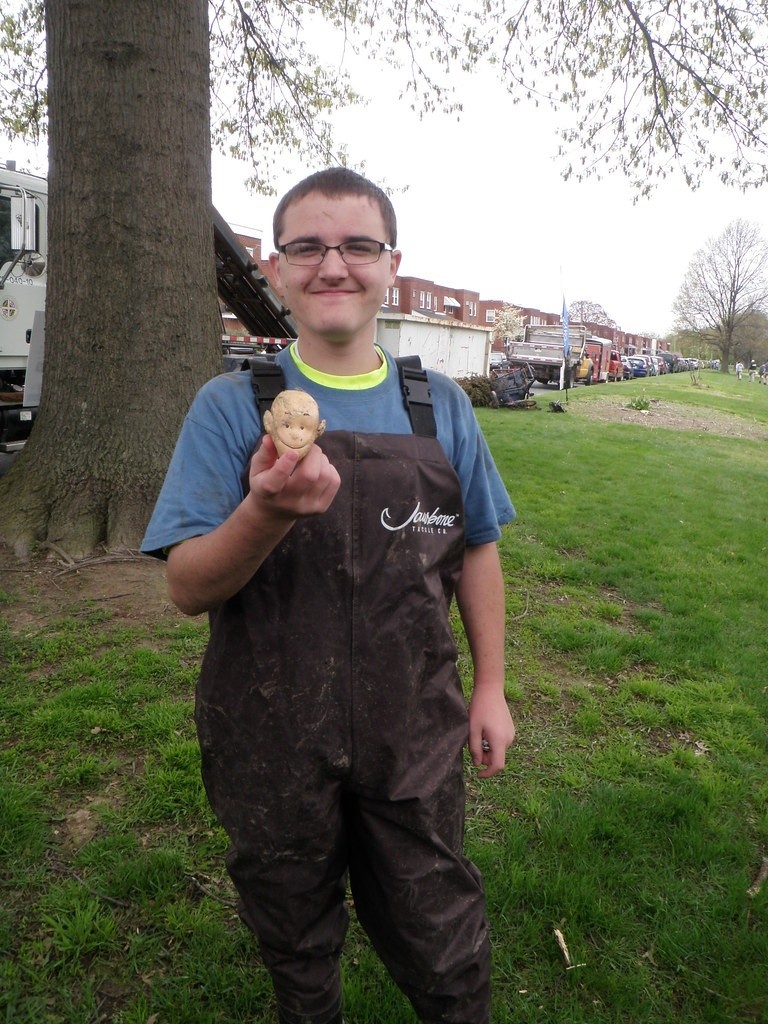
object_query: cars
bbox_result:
[607,348,720,383]
[490,353,511,370]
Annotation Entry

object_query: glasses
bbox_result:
[278,238,393,266]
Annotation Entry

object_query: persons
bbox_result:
[714,361,719,370]
[748,360,757,383]
[759,360,768,384]
[141,168,516,1024]
[736,361,744,380]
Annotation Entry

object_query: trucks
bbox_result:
[585,336,612,385]
[508,324,597,389]
[0,164,302,453]
